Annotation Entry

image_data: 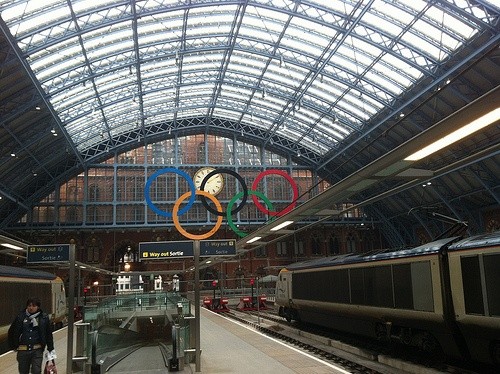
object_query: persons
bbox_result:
[8,297,54,374]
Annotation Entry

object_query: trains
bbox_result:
[0,264,69,354]
[272,233,500,368]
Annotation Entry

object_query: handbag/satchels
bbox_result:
[44,350,57,374]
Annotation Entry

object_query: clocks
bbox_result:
[192,167,225,197]
